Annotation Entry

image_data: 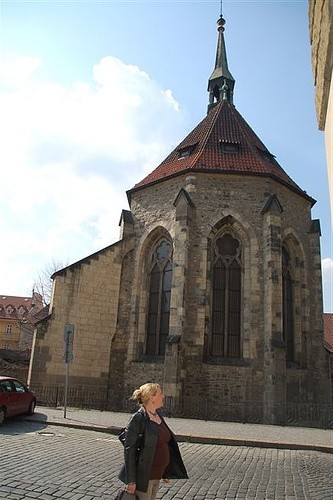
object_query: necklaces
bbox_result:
[145,407,158,417]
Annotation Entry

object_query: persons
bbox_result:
[117,381,190,500]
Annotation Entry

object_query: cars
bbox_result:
[0,376,36,429]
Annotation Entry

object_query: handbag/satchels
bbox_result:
[113,489,138,500]
[119,428,145,453]
